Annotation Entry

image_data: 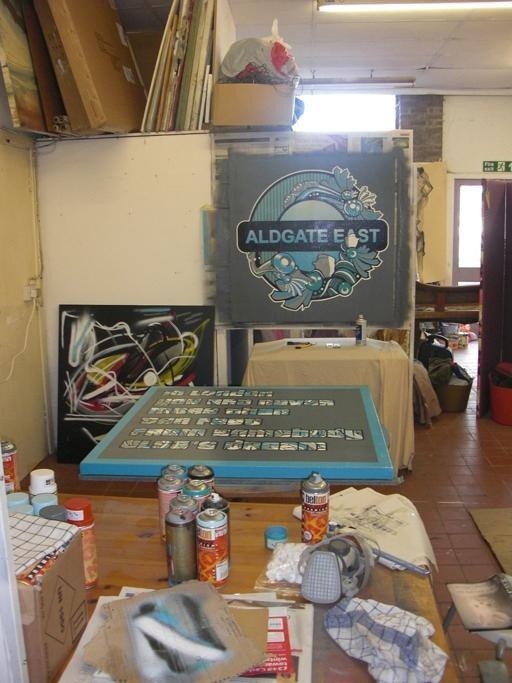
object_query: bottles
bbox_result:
[1,436,101,588]
[355,315,367,344]
[298,472,330,543]
[156,464,231,585]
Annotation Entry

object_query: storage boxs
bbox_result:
[209,78,297,127]
[430,378,471,413]
[7,512,89,681]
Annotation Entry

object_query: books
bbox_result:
[140,0,213,132]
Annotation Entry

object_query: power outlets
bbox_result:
[24,284,36,302]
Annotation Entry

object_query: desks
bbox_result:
[7,489,458,682]
[79,382,397,502]
[241,335,417,479]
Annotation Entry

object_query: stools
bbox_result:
[441,573,512,664]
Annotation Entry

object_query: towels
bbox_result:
[320,589,451,681]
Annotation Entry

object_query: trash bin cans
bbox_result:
[488,361,512,426]
